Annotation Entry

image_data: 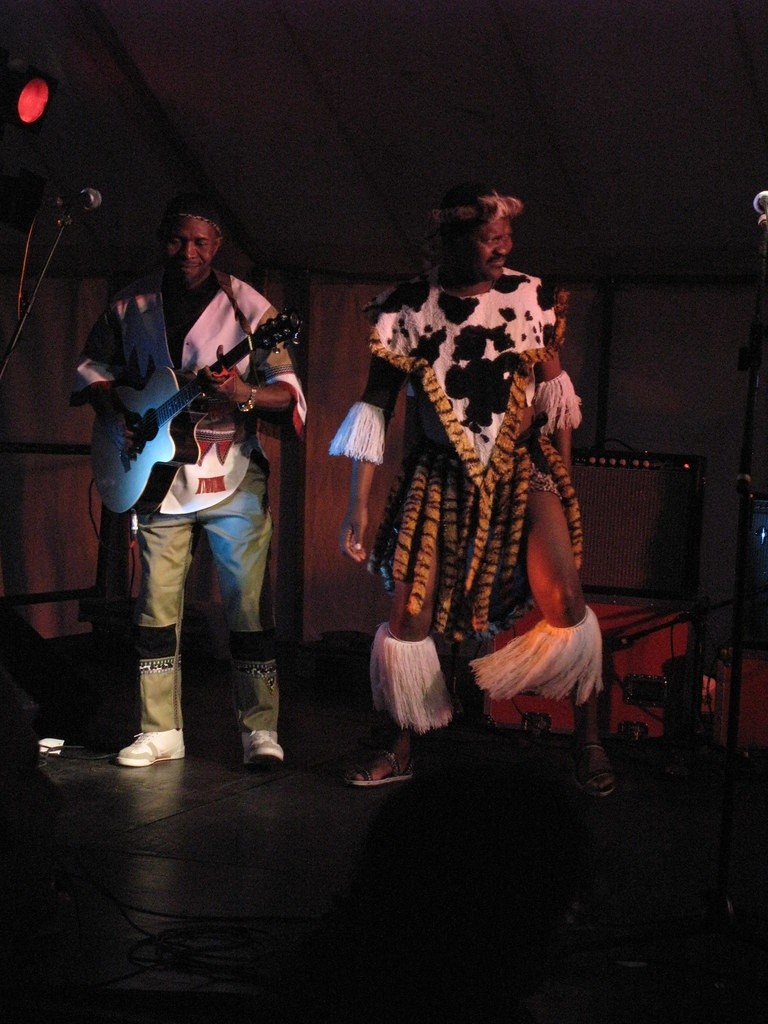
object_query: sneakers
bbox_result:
[241,731,285,765]
[117,728,186,766]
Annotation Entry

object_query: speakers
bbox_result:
[569,448,704,598]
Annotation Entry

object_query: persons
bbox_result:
[327,184,617,797]
[66,197,307,770]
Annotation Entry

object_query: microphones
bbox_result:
[45,187,103,211]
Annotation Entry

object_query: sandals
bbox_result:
[576,741,615,798]
[344,748,414,786]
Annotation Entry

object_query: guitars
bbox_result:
[91,305,300,514]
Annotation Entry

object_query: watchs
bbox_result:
[238,385,257,412]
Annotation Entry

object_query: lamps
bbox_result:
[0,64,57,136]
[0,167,48,234]
